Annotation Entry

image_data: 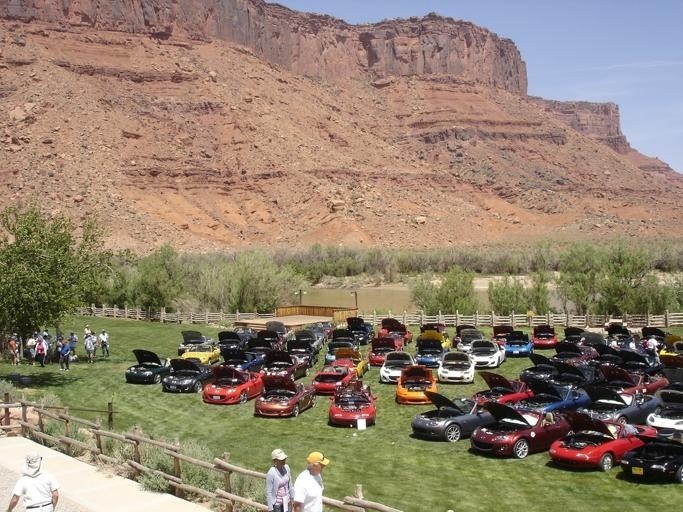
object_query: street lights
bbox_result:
[294,289,307,305]
[350,291,358,308]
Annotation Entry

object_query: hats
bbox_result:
[272,448,288,460]
[26,455,41,468]
[307,452,329,465]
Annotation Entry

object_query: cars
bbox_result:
[618,432,683,483]
[504,330,536,358]
[252,375,316,418]
[469,402,572,459]
[548,409,658,473]
[211,317,485,396]
[161,358,211,394]
[181,344,220,365]
[410,390,501,444]
[466,339,506,369]
[125,349,170,386]
[328,379,378,428]
[490,325,514,346]
[470,371,534,407]
[512,321,683,437]
[178,330,215,356]
[201,365,265,405]
[395,365,439,406]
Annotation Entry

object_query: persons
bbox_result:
[291,452,330,512]
[6,451,58,512]
[266,449,294,512]
[8,325,110,372]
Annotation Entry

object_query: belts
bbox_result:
[27,503,51,508]
